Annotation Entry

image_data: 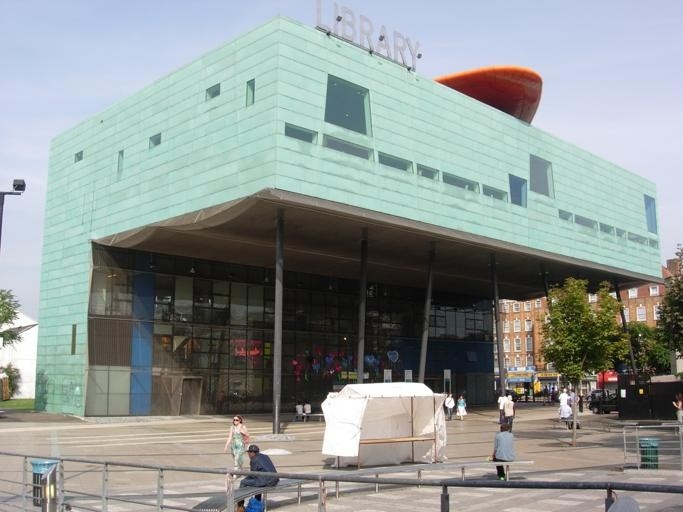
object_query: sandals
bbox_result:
[232,477,237,481]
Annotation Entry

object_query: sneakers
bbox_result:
[236,506,245,512]
[486,456,492,463]
[497,476,504,480]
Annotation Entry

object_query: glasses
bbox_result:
[233,419,239,422]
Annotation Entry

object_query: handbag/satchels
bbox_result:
[246,498,264,512]
[445,407,447,410]
[242,434,250,445]
[456,412,459,417]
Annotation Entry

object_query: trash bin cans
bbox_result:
[639,438,658,468]
[31,460,59,507]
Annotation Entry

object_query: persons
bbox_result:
[234,445,280,512]
[672,392,683,436]
[542,383,584,429]
[502,395,515,433]
[492,423,515,481]
[304,400,312,422]
[224,414,250,480]
[455,393,468,421]
[445,393,455,421]
[497,391,509,424]
[295,400,304,422]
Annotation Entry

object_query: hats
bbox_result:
[247,445,259,452]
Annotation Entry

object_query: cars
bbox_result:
[585,389,618,415]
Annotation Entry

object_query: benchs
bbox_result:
[187,458,533,512]
[294,413,324,423]
